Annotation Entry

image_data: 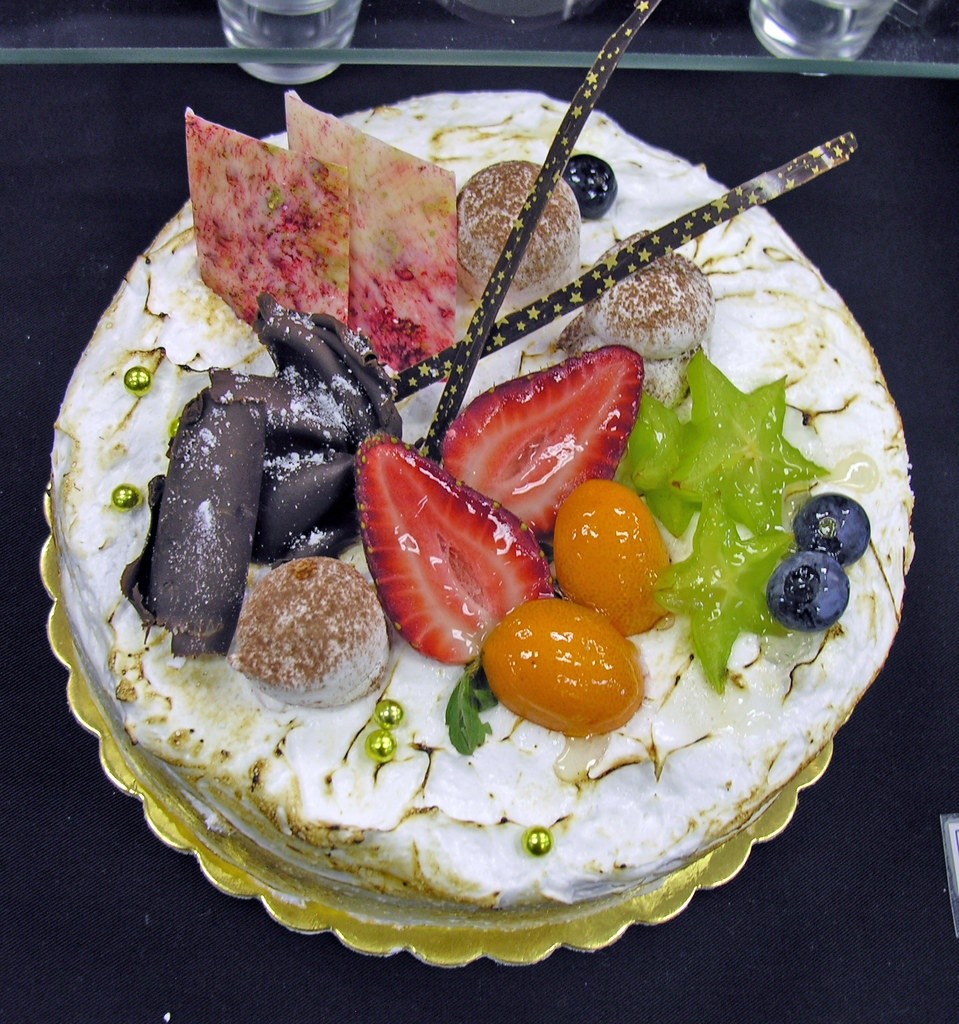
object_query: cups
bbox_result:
[219,0,362,85]
[749,0,896,76]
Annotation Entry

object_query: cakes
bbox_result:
[48,89,915,909]
